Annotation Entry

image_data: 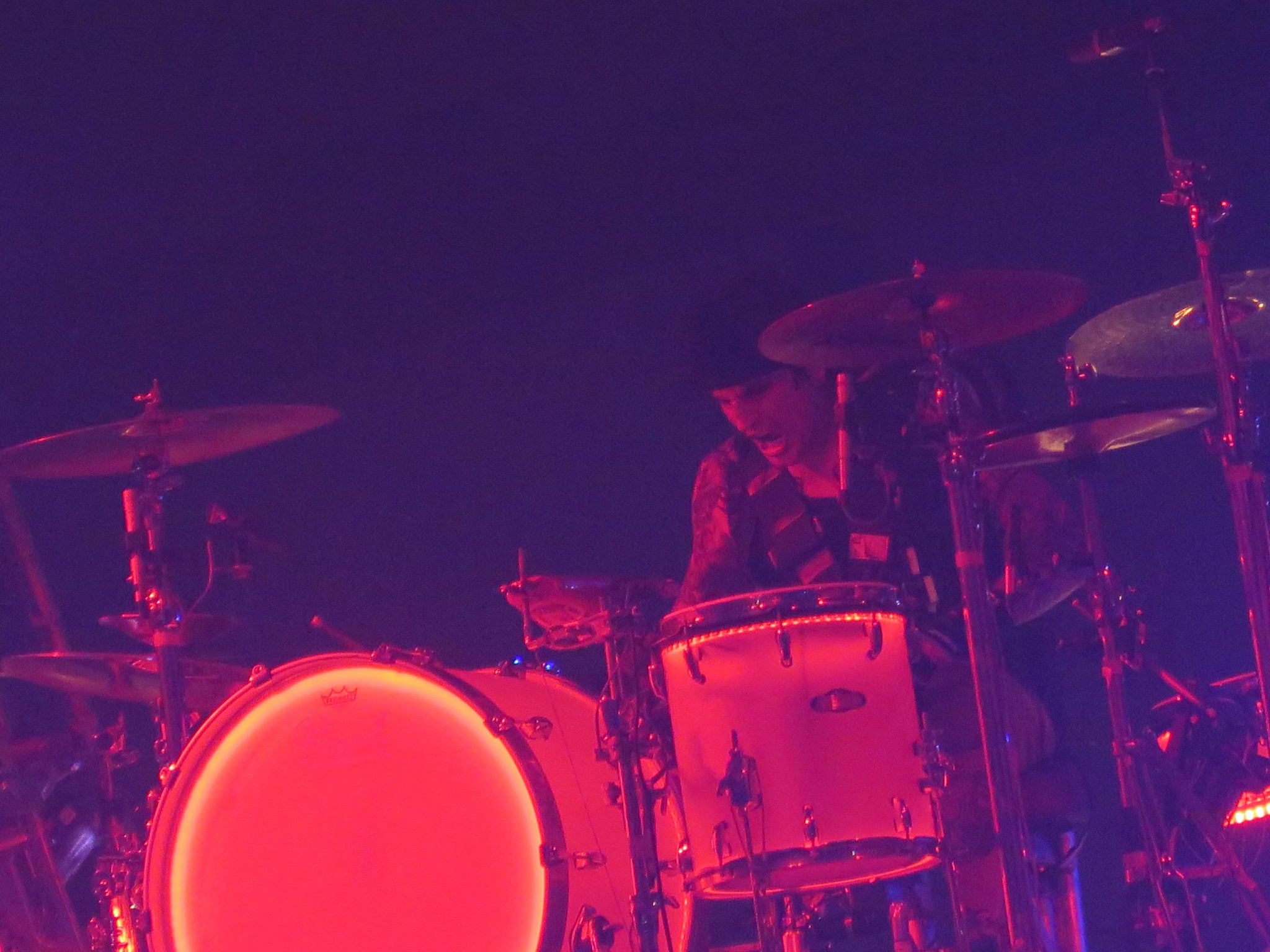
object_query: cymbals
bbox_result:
[955,399,1218,473]
[0,647,249,712]
[754,265,1089,374]
[1066,266,1267,382]
[0,397,342,481]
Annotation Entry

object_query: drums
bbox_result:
[136,649,691,945]
[642,582,954,898]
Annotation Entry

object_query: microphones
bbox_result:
[834,372,850,493]
[1068,16,1167,62]
[160,476,280,558]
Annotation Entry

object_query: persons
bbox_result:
[646,269,1075,951]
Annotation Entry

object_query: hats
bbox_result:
[695,287,800,391]
